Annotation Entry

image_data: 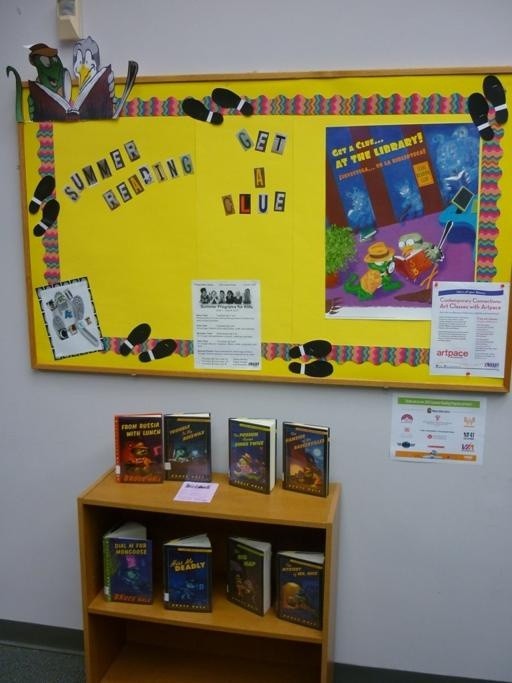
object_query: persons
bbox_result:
[200,288,250,304]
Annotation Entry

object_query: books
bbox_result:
[228,417,278,494]
[113,413,165,483]
[283,421,329,497]
[226,535,272,616]
[103,521,154,605]
[163,531,214,613]
[276,550,325,630]
[163,412,213,482]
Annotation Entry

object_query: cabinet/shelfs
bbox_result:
[74,465,345,677]
